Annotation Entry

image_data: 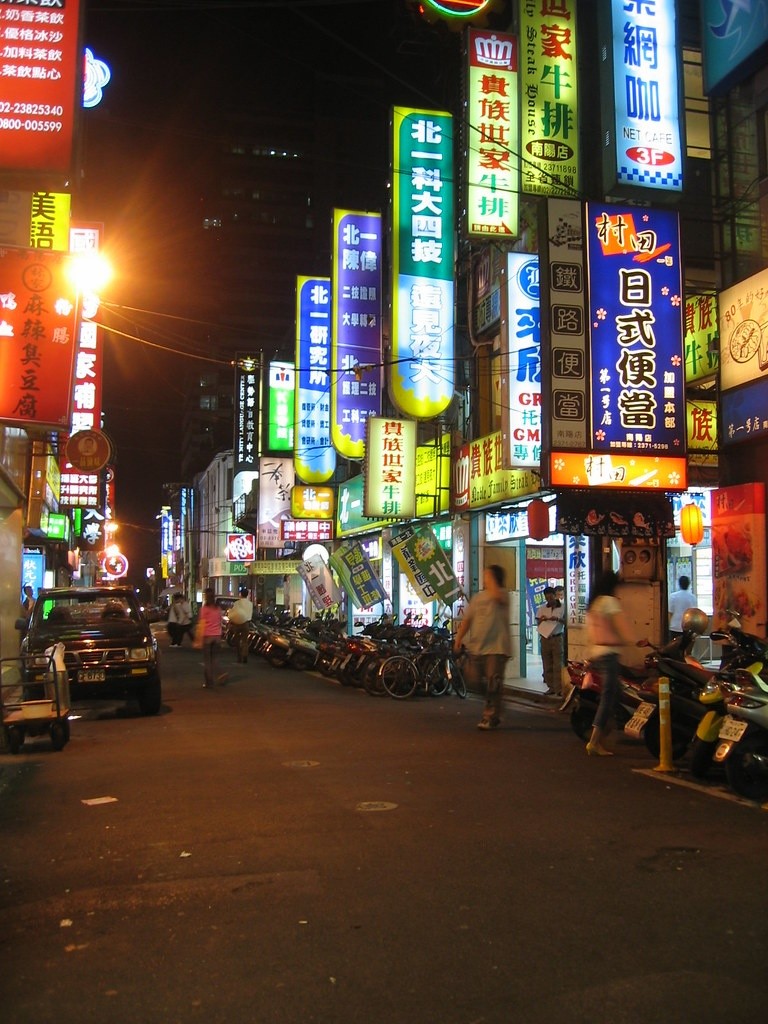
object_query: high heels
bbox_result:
[586,741,612,757]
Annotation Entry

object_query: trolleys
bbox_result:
[0,654,72,754]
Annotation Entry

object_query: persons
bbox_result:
[22,585,253,688]
[535,576,697,756]
[454,564,512,728]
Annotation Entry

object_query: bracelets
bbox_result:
[557,617,560,621]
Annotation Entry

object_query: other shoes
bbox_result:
[243,657,247,663]
[203,683,213,689]
[543,689,555,695]
[237,657,242,663]
[478,719,499,729]
[558,692,563,696]
[220,676,227,686]
[169,643,183,648]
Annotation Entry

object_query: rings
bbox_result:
[542,618,544,619]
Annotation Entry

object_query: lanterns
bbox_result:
[681,504,703,545]
[528,499,549,541]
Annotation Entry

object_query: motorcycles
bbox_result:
[221,597,466,701]
[557,605,767,803]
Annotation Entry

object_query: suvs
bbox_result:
[14,585,163,716]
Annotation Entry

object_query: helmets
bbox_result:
[682,608,709,634]
